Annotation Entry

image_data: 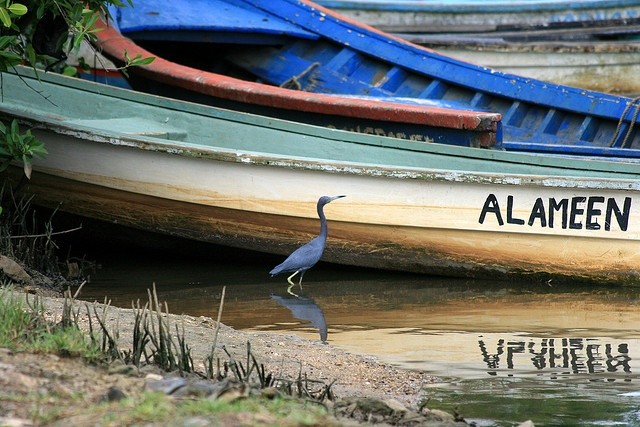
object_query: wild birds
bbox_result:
[269,195,345,286]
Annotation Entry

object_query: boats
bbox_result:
[0,0,640,289]
[306,0,640,97]
[79,0,640,160]
